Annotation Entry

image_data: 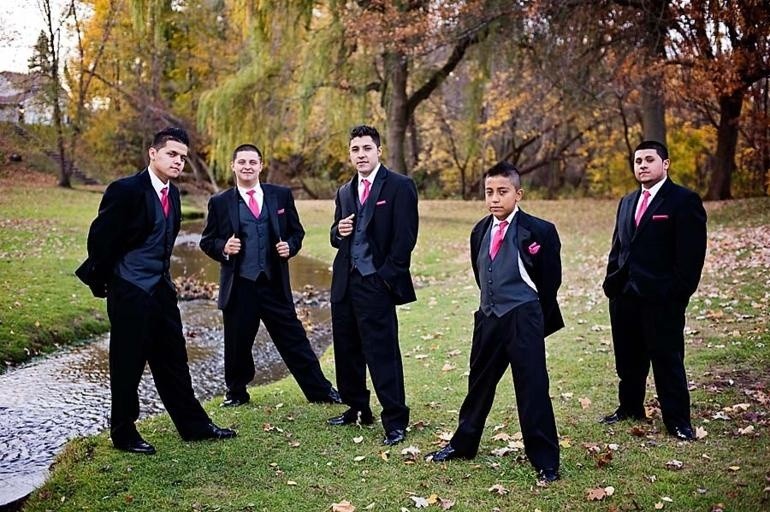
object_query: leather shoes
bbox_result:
[326,387,342,403]
[126,439,157,455]
[540,467,559,481]
[383,428,409,447]
[220,399,249,407]
[326,413,373,426]
[669,426,697,441]
[424,443,457,462]
[185,422,236,442]
[599,410,649,426]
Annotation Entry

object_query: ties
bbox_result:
[160,187,169,218]
[361,179,371,205]
[491,221,509,257]
[635,191,651,226]
[246,190,259,220]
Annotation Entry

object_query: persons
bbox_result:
[423,161,565,482]
[602,141,707,440]
[326,126,419,443]
[74,127,237,454]
[199,144,343,407]
